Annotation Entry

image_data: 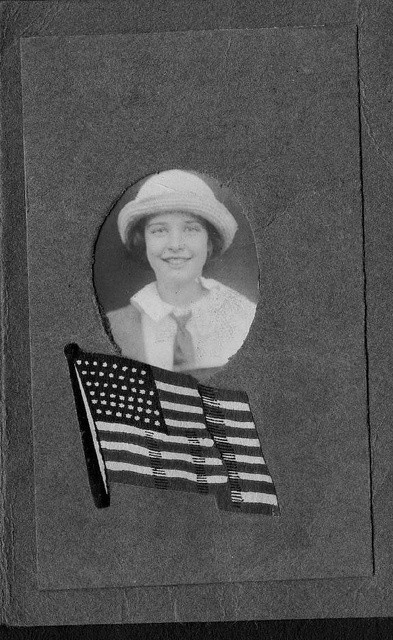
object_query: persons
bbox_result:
[106,167,256,371]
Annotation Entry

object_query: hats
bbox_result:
[116,171,239,257]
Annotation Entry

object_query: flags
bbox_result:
[68,346,279,515]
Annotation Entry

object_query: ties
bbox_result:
[170,310,194,372]
